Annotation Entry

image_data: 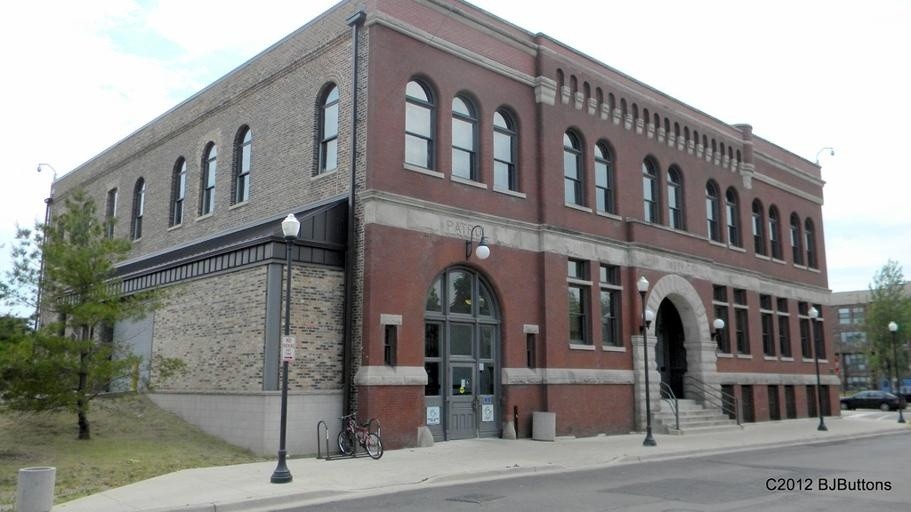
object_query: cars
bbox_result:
[840,390,906,412]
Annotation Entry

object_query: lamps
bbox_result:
[710,318,724,340]
[639,309,654,336]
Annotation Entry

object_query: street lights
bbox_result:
[270,213,300,484]
[890,322,907,424]
[808,307,827,431]
[30,162,58,326]
[637,276,657,446]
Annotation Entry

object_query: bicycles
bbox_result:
[336,413,383,461]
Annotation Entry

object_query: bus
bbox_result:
[882,378,911,396]
